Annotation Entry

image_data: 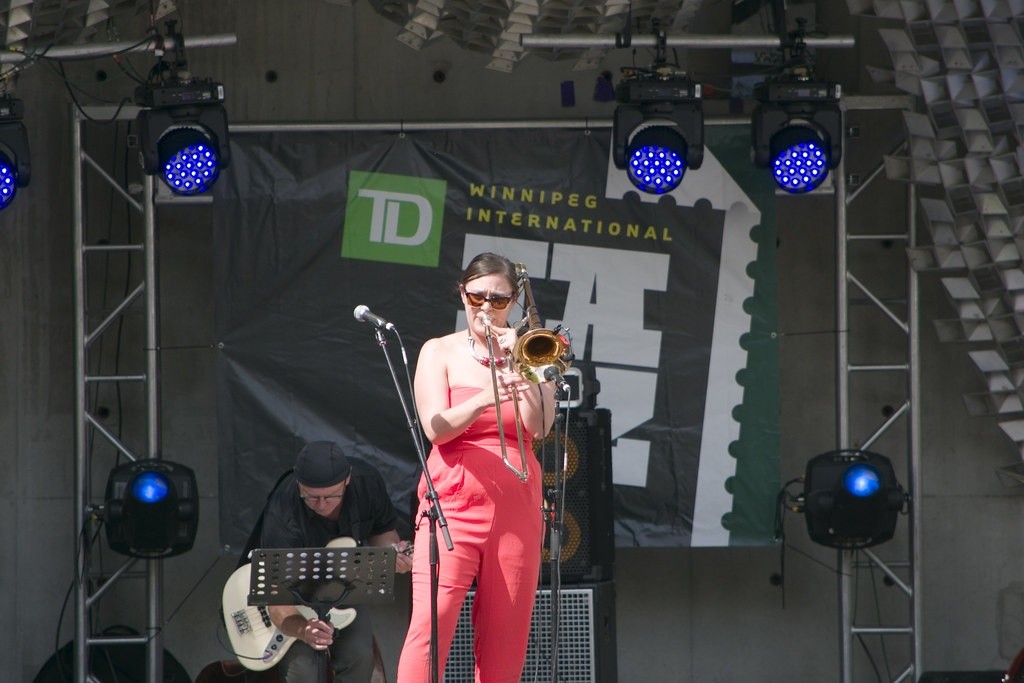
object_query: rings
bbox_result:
[500,336,506,343]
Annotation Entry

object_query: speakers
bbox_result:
[469,408,614,589]
[442,580,618,683]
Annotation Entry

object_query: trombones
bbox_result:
[482,263,571,484]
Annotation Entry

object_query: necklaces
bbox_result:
[468,331,506,366]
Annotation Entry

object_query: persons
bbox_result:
[396,252,555,683]
[259,441,414,683]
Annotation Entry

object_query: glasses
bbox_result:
[296,478,347,503]
[463,281,514,310]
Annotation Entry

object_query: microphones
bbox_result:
[543,366,571,392]
[354,305,395,331]
[563,353,575,362]
[553,325,562,335]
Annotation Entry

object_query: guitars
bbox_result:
[222,536,415,671]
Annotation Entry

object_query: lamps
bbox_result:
[0,120,32,211]
[101,459,201,562]
[135,73,232,197]
[613,20,705,195]
[804,448,912,549]
[749,28,842,195]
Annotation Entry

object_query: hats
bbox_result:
[294,441,351,488]
